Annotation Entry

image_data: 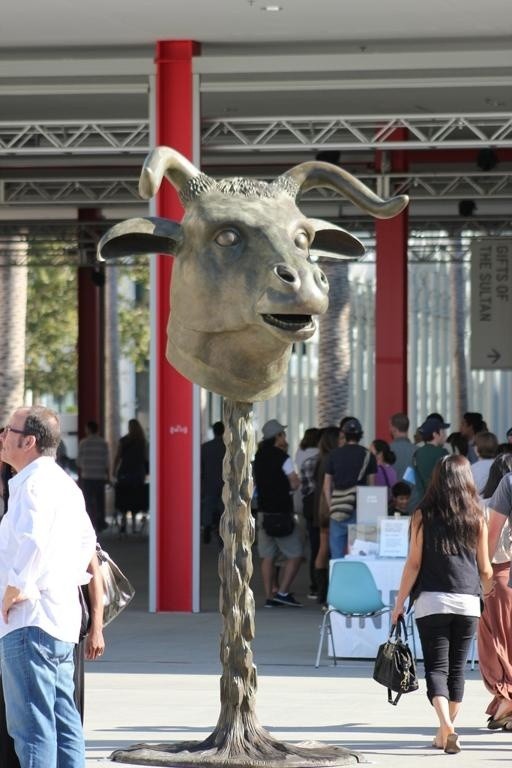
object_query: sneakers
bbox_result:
[308,591,317,599]
[264,600,277,608]
[273,594,302,607]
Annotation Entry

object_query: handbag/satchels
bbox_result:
[330,450,371,520]
[373,615,418,705]
[79,540,135,640]
[254,459,294,537]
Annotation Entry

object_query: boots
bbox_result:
[315,569,328,603]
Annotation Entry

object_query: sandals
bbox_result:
[433,738,443,748]
[444,729,461,754]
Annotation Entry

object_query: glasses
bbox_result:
[4,425,24,435]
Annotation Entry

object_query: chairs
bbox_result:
[403,607,478,671]
[314,561,395,668]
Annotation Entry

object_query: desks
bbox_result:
[330,556,481,662]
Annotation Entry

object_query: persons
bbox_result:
[314,427,346,604]
[391,455,493,754]
[302,453,318,600]
[0,406,96,768]
[389,413,420,483]
[388,482,410,516]
[368,439,397,504]
[254,419,305,608]
[478,453,512,732]
[112,419,148,533]
[76,422,112,531]
[408,417,450,515]
[300,452,322,599]
[0,428,105,768]
[488,471,512,588]
[295,428,320,471]
[470,432,498,499]
[460,412,483,465]
[201,422,226,548]
[323,417,378,559]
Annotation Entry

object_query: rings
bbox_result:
[97,653,101,657]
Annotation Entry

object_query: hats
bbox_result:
[422,419,451,435]
[262,420,288,439]
[343,419,362,433]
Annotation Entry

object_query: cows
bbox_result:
[94,146,411,404]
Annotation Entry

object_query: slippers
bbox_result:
[488,713,511,729]
[502,722,512,732]
